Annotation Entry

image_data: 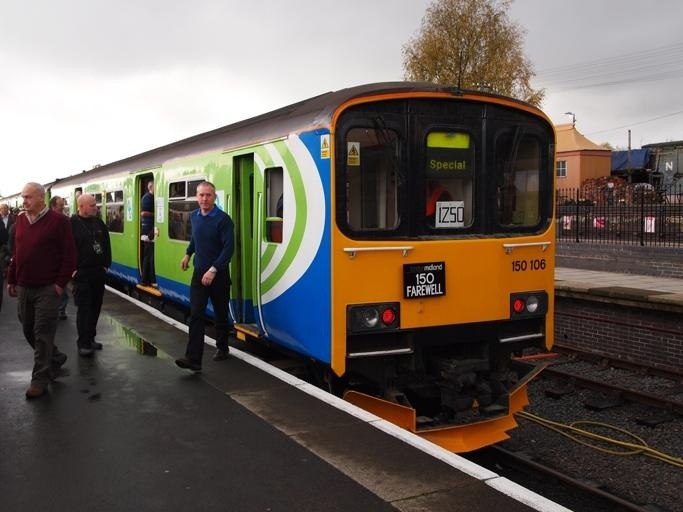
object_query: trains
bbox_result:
[0,78,564,459]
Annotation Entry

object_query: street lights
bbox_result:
[564,111,576,127]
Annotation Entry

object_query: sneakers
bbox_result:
[79,341,103,356]
[49,353,66,381]
[25,386,48,398]
[213,348,229,361]
[175,357,202,371]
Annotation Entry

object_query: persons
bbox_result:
[426,177,452,225]
[1,194,112,357]
[138,177,156,288]
[173,180,234,371]
[498,184,516,225]
[5,183,79,398]
